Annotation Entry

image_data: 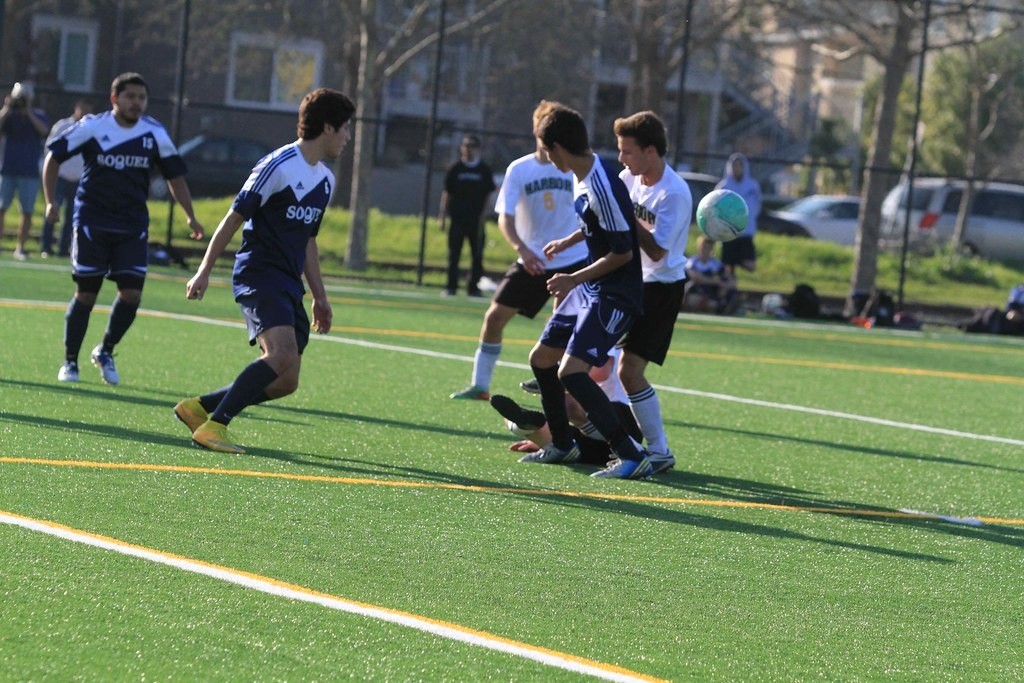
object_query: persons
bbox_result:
[174,89,356,453]
[716,153,761,273]
[614,112,692,474]
[683,236,727,312]
[0,83,49,259]
[491,346,643,468]
[438,136,495,298]
[42,74,203,384]
[449,100,589,401]
[40,99,94,258]
[517,107,653,480]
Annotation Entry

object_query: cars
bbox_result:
[878,177,1024,269]
[674,170,813,241]
[774,194,862,247]
[147,133,278,205]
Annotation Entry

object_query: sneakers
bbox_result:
[591,453,653,480]
[490,394,546,430]
[191,420,245,454]
[174,396,208,433]
[520,379,541,393]
[518,445,582,463]
[58,363,78,381]
[645,450,676,474]
[90,347,119,385]
[448,387,490,401]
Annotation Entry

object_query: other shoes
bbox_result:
[12,250,29,259]
[40,248,54,257]
[56,249,70,257]
[446,287,455,297]
[468,288,485,297]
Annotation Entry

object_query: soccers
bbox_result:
[696,188,749,242]
